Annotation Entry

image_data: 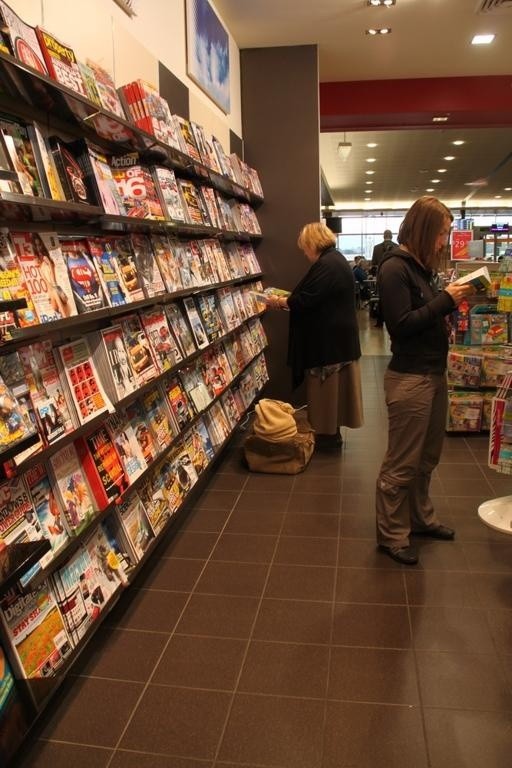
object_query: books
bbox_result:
[250,286,291,312]
[0,0,272,764]
[453,266,492,291]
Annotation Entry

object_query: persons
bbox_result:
[353,229,398,329]
[265,221,364,451]
[376,195,472,565]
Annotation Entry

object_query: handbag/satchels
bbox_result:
[240,398,315,475]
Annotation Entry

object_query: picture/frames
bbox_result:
[183,1,234,116]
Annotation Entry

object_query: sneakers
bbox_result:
[412,524,454,538]
[379,544,418,565]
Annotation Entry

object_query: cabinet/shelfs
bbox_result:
[2,45,276,768]
